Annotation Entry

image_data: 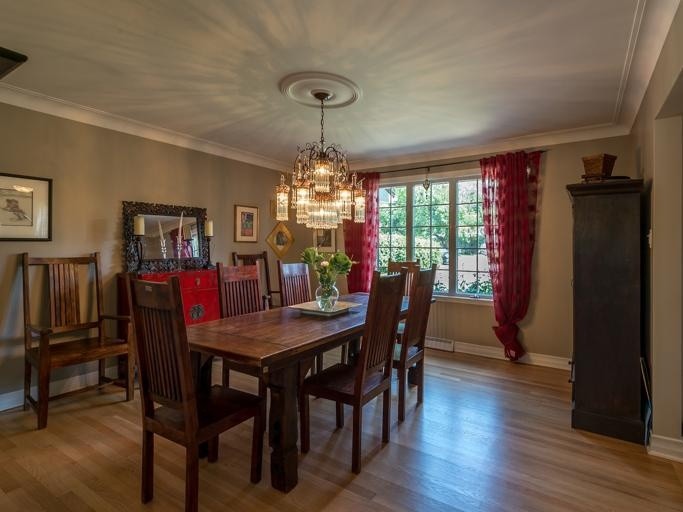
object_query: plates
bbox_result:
[287,300,363,317]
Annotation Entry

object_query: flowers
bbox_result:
[299,248,352,309]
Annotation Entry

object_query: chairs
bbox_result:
[300,266,409,476]
[124,271,266,512]
[275,260,347,399]
[351,258,421,378]
[348,263,438,422]
[214,259,269,429]
[231,250,283,310]
[20,250,135,430]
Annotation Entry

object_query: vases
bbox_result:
[315,282,339,312]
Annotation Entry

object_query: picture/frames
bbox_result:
[0,172,54,241]
[312,228,336,254]
[266,222,294,260]
[233,204,258,243]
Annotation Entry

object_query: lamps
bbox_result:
[272,71,367,231]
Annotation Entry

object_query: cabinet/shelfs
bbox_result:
[563,179,646,446]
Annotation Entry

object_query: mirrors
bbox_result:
[121,200,210,271]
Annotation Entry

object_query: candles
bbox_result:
[177,211,183,237]
[133,215,144,234]
[183,224,192,240]
[204,219,213,236]
[157,220,164,241]
